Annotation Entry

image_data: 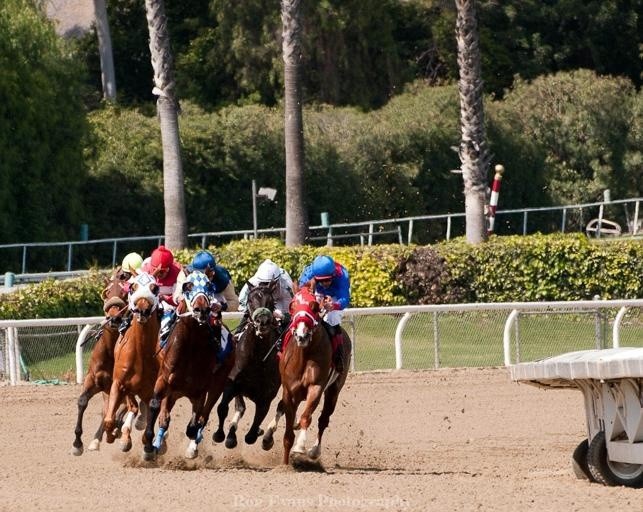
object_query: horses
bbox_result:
[213,269,295,452]
[276,274,356,477]
[67,261,232,466]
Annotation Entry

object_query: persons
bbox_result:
[158,249,241,377]
[234,258,297,343]
[139,243,187,315]
[275,255,351,378]
[116,252,144,288]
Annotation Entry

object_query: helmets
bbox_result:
[256,259,280,282]
[193,252,215,270]
[150,245,173,268]
[121,252,143,274]
[312,255,334,279]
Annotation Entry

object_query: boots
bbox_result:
[330,325,344,372]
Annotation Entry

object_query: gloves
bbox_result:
[211,303,221,312]
[273,310,281,322]
[322,296,333,311]
